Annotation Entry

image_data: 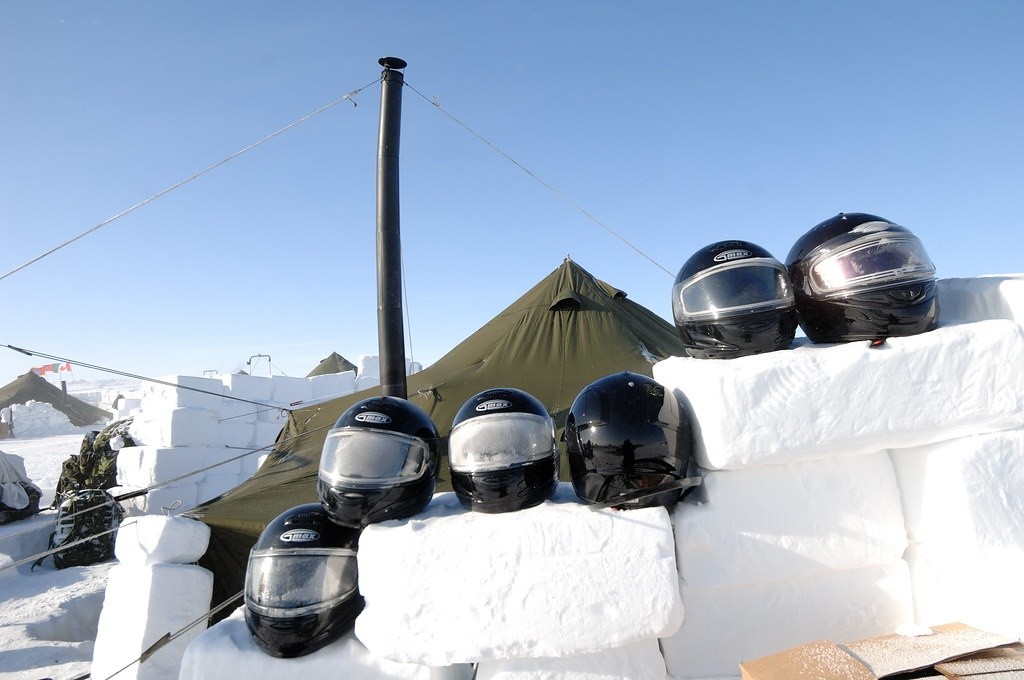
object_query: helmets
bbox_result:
[568,371,704,509]
[245,502,365,660]
[447,387,561,513]
[786,212,941,346]
[316,396,442,527]
[672,241,797,358]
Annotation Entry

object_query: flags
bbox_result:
[32,368,44,375]
[60,363,70,372]
[43,363,58,372]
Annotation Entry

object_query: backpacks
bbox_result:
[52,417,134,508]
[31,489,127,570]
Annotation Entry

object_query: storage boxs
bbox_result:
[739,622,1024,680]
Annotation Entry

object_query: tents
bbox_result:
[307,353,358,377]
[187,261,687,625]
